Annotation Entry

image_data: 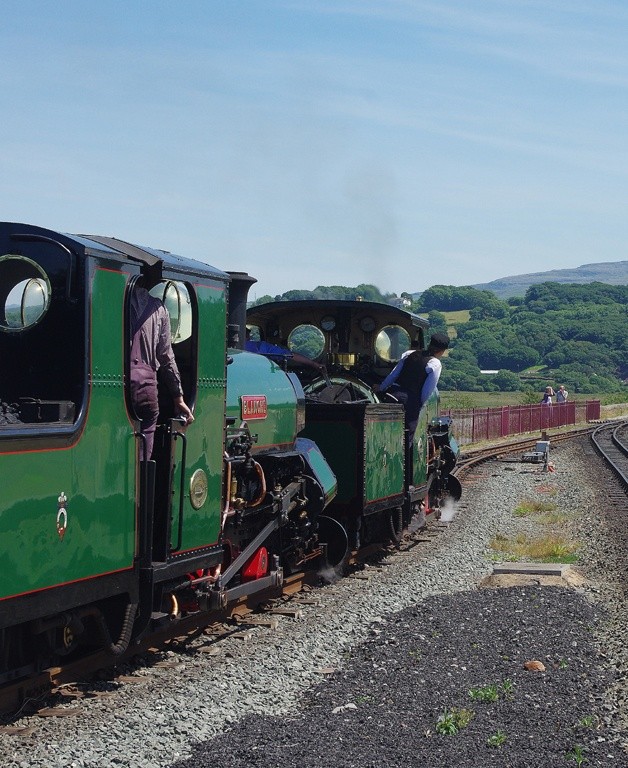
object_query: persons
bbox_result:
[556,385,568,403]
[376,333,451,450]
[542,386,555,404]
[130,274,195,462]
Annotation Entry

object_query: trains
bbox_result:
[0,220,463,686]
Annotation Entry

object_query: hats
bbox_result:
[430,333,450,349]
